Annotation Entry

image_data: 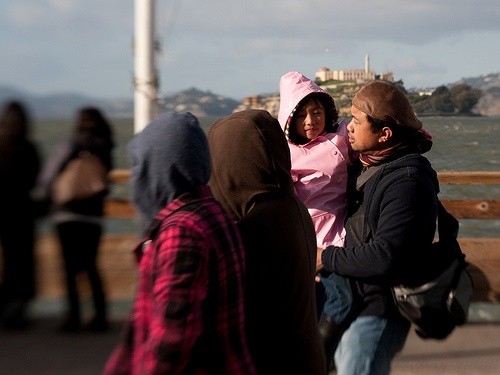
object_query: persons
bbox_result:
[317,80,440,375]
[278,71,359,369]
[208,110,326,375]
[0,99,44,328]
[103,112,257,375]
[39,105,118,333]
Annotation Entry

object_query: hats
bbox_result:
[352,80,423,130]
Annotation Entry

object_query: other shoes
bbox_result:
[57,316,81,334]
[86,315,110,333]
[319,316,346,375]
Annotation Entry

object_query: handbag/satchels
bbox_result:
[368,169,475,342]
[50,136,106,205]
